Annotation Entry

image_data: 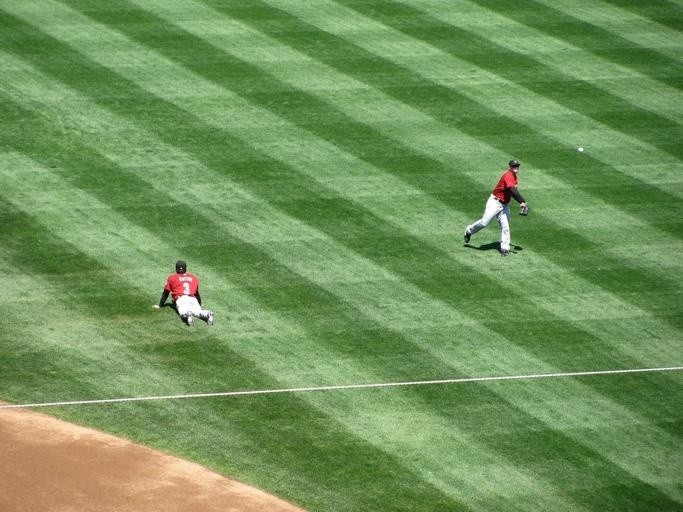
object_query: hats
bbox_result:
[175,260,186,274]
[509,159,520,168]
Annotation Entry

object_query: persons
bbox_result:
[151,260,214,327]
[463,159,527,256]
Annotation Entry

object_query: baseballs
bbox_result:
[578,147,583,152]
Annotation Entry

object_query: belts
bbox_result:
[494,197,506,205]
[174,295,194,301]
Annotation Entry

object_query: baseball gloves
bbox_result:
[519,205,528,216]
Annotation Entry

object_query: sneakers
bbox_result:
[500,249,509,257]
[206,311,214,326]
[463,230,470,243]
[186,311,194,326]
